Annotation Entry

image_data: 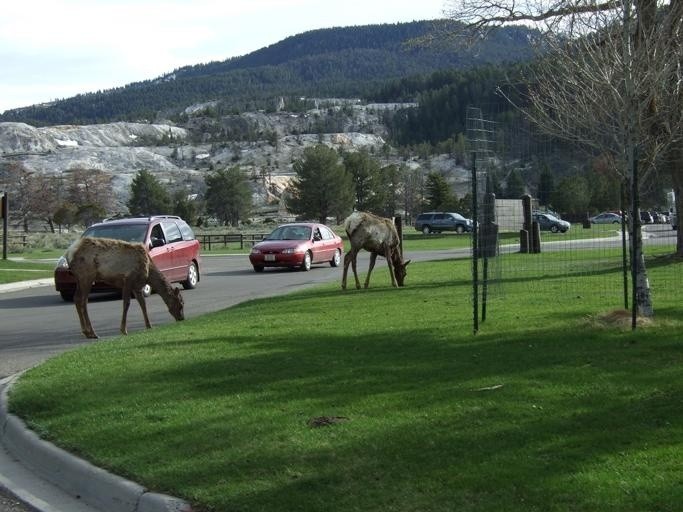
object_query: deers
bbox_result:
[65,236,186,340]
[340,209,411,290]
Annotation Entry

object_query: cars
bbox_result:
[591,208,666,224]
[249,223,344,272]
[532,213,570,233]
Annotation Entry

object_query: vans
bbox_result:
[415,212,479,234]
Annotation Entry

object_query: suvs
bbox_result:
[55,215,200,302]
